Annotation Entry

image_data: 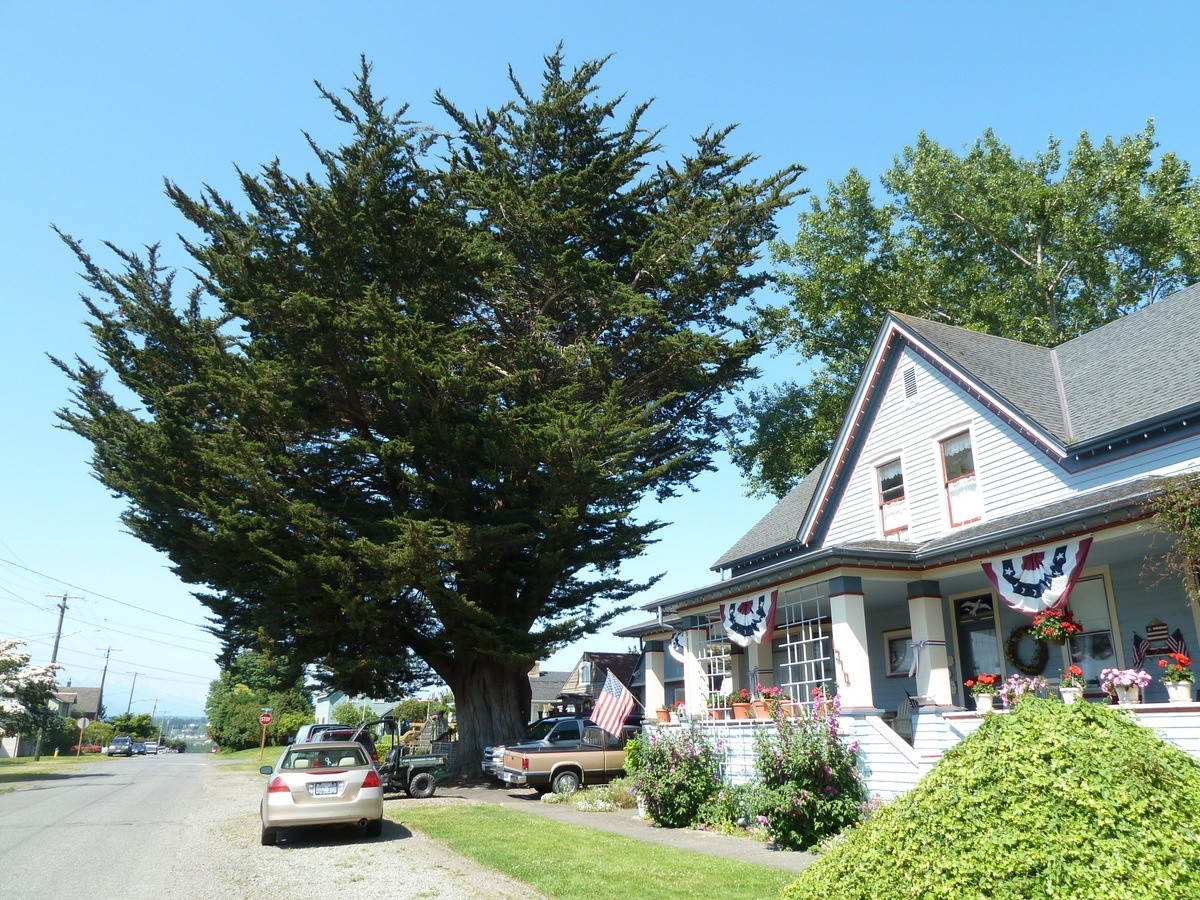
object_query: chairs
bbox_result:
[339,757,356,766]
[377,749,399,771]
[295,758,310,768]
[884,699,913,741]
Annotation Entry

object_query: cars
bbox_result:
[133,742,146,755]
[70,742,101,754]
[258,740,384,846]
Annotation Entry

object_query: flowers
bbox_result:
[1156,654,1195,683]
[811,686,832,698]
[1099,665,1151,697]
[1028,609,1083,638]
[670,700,684,711]
[1060,666,1085,686]
[732,688,751,702]
[964,671,997,692]
[1000,672,1046,705]
[756,685,782,698]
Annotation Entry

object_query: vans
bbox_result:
[144,742,178,755]
[285,722,380,773]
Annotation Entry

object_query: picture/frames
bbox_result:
[882,627,916,679]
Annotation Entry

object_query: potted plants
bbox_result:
[779,694,797,716]
[657,705,669,724]
[707,691,728,720]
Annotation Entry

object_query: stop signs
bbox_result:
[259,713,273,726]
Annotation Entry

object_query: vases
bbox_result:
[1164,679,1194,704]
[754,701,774,718]
[1113,685,1142,705]
[815,700,830,719]
[1050,631,1066,647]
[732,703,750,720]
[1061,689,1084,703]
[669,712,680,724]
[972,694,992,710]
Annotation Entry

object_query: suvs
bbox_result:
[107,735,133,756]
[481,715,598,778]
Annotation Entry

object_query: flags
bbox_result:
[1132,633,1150,672]
[589,669,635,739]
[1165,628,1190,658]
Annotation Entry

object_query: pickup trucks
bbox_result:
[497,724,643,796]
